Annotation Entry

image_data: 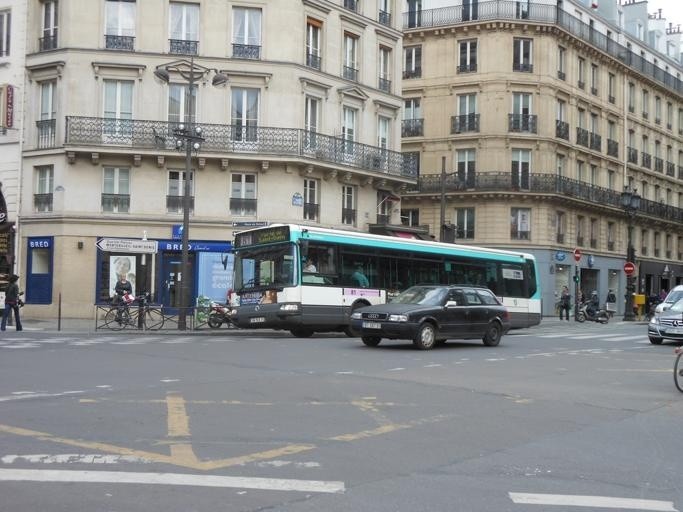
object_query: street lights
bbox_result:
[154,56,229,330]
[620,185,642,321]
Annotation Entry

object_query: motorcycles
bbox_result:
[208,300,237,328]
[575,298,611,324]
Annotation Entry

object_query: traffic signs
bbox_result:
[94,238,158,254]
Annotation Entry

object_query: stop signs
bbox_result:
[623,261,636,275]
[573,247,583,262]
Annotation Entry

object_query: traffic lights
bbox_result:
[573,274,580,283]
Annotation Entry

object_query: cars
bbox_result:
[348,282,513,350]
[647,284,683,345]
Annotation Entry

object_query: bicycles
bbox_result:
[673,348,683,393]
[104,296,165,330]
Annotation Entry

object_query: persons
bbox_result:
[109,256,130,296]
[588,290,599,310]
[114,272,131,319]
[605,289,616,317]
[557,285,572,321]
[352,265,369,288]
[1,274,24,331]
[304,256,318,272]
[578,289,584,302]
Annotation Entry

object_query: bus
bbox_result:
[220,222,544,337]
